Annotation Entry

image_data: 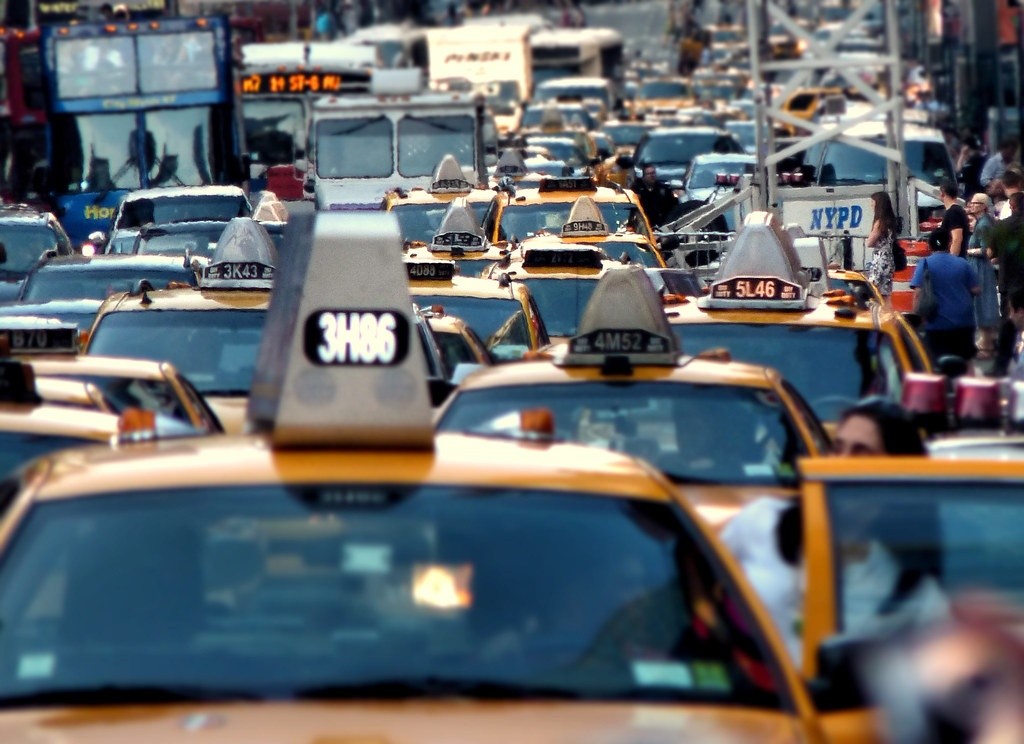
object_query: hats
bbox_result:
[923,227,953,246]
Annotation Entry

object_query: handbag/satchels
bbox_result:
[891,229,908,272]
[912,256,939,323]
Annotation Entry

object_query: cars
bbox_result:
[0,208,1024,744]
[2,6,1024,541]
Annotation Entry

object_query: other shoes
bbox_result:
[977,344,998,358]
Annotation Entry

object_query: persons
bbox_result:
[1006,288,1024,377]
[718,398,946,665]
[965,193,999,360]
[627,164,680,234]
[939,1,1024,377]
[867,192,901,303]
[911,225,984,376]
[210,0,714,74]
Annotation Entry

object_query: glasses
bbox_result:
[967,202,985,205]
